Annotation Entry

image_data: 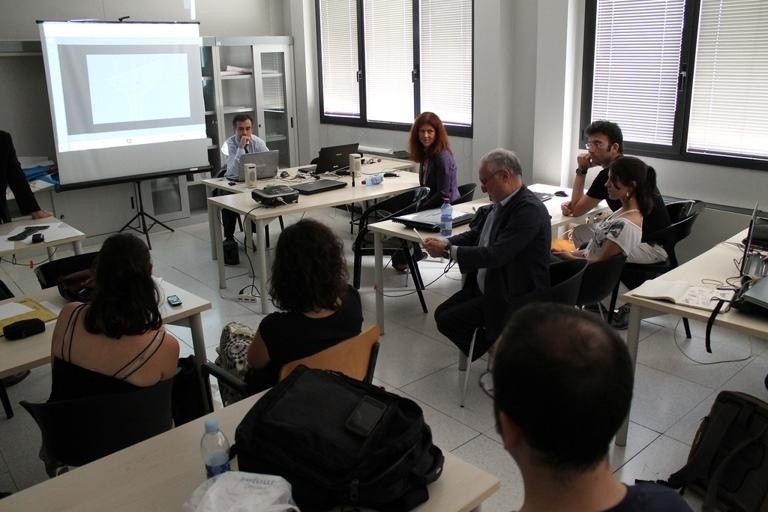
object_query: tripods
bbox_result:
[115,180,175,250]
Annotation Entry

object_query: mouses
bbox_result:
[384,171,400,177]
[554,191,568,196]
[281,170,289,178]
[31,232,44,244]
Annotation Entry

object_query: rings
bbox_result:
[427,250,430,254]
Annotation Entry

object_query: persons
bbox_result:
[215,219,362,407]
[51,234,180,477]
[421,147,552,362]
[220,114,270,252]
[551,158,657,264]
[0,130,53,224]
[561,121,670,331]
[391,112,461,271]
[492,302,694,512]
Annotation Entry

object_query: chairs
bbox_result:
[607,200,706,339]
[420,183,478,211]
[19,354,200,478]
[353,187,431,314]
[202,324,381,409]
[458,260,591,408]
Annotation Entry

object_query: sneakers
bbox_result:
[244,238,256,252]
[612,303,632,330]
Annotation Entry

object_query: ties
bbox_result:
[243,145,248,153]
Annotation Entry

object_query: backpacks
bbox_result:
[233,364,445,511]
[669,391,768,511]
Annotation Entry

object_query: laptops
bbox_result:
[291,179,348,194]
[740,199,767,279]
[227,148,280,182]
[298,142,360,175]
[532,191,553,202]
[391,209,474,232]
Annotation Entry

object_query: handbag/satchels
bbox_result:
[58,269,97,301]
[3,318,45,341]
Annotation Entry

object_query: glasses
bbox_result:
[586,142,612,149]
[479,372,497,401]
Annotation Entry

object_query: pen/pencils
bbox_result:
[413,227,424,244]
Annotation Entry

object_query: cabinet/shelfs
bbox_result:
[138,35,302,222]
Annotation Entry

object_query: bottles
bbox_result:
[361,176,383,185]
[200,419,229,478]
[376,159,384,181]
[440,198,452,235]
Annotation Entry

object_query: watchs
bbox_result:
[575,168,587,175]
[442,244,452,260]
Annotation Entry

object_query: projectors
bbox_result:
[250,185,299,207]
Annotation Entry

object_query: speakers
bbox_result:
[245,164,257,187]
[348,153,362,175]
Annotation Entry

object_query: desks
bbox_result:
[0,215,87,258]
[615,228,766,447]
[368,183,615,371]
[1,387,501,512]
[1,275,213,419]
[201,154,421,313]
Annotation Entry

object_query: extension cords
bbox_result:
[235,295,256,302]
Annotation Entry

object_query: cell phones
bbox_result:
[167,294,183,306]
[413,228,428,246]
